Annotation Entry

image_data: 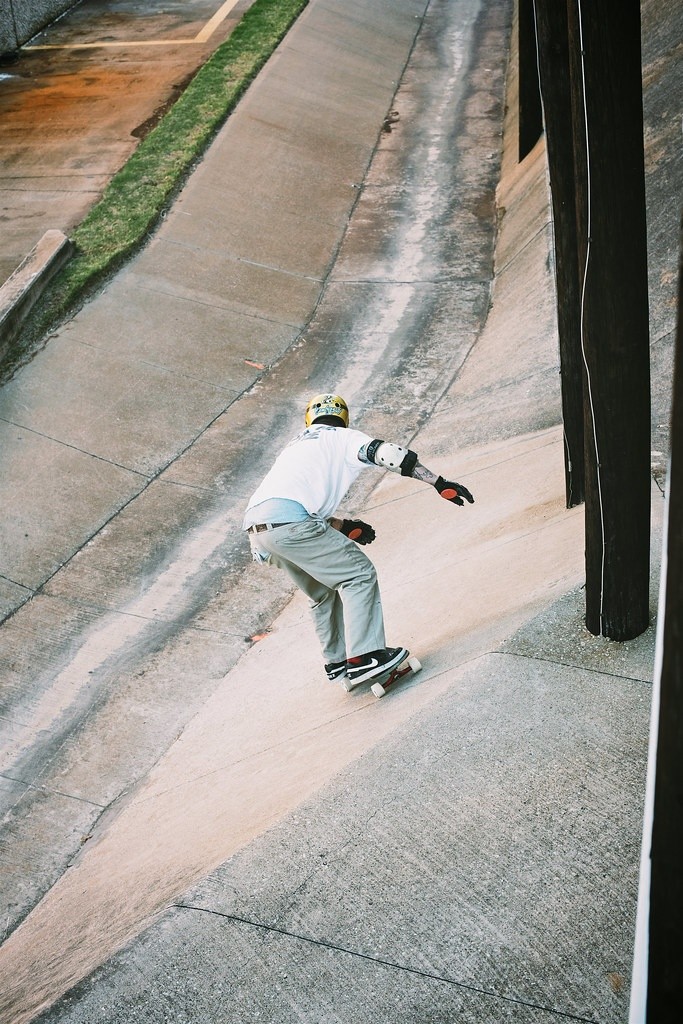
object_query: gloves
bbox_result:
[340,520,376,545]
[434,475,475,506]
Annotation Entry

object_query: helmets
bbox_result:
[305,394,349,427]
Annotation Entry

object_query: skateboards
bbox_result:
[340,649,424,700]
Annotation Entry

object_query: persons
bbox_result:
[242,394,475,685]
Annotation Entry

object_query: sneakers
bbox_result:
[324,659,346,684]
[346,646,409,686]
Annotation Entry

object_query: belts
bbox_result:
[247,522,291,534]
